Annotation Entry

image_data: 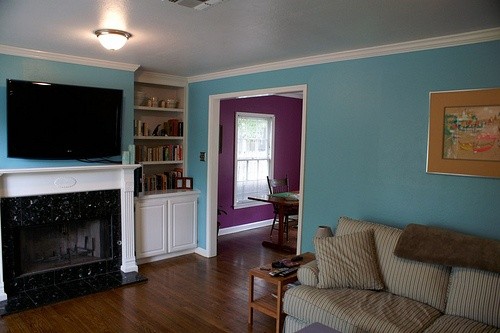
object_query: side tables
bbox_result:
[247,251,316,333]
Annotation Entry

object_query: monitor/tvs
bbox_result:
[5,79,123,160]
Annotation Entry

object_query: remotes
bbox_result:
[268,267,290,277]
[278,268,298,276]
[290,256,304,262]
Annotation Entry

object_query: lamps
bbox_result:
[95,28,133,51]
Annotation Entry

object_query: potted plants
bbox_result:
[217,205,228,237]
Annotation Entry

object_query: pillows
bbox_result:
[313,229,385,290]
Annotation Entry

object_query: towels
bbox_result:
[393,223,500,273]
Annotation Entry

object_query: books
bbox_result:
[272,287,289,300]
[134,119,149,136]
[143,167,183,191]
[136,143,183,162]
[164,119,183,136]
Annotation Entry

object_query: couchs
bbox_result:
[283,216,500,333]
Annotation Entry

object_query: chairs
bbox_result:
[266,173,290,235]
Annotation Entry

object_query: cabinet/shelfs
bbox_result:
[134,194,201,266]
[134,80,189,192]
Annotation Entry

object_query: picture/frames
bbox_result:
[425,87,500,178]
